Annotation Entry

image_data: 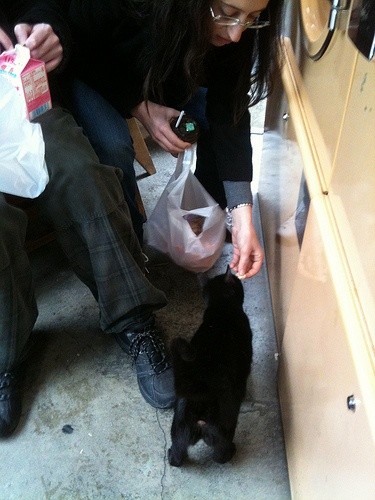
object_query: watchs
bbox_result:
[226,203,254,216]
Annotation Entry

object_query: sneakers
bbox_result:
[0,369,22,437]
[114,315,177,408]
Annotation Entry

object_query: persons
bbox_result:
[0,0,286,439]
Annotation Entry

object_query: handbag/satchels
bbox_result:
[143,145,226,273]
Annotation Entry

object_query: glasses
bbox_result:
[209,5,271,29]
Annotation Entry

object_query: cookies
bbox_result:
[235,272,246,280]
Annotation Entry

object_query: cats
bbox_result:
[167,263,253,468]
